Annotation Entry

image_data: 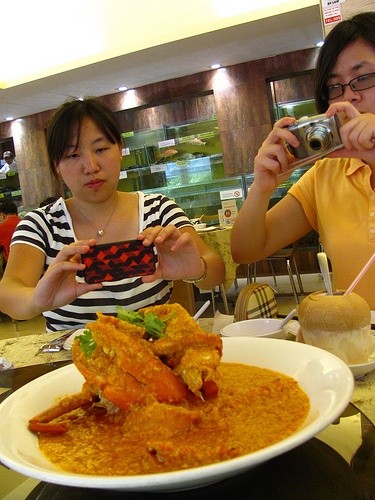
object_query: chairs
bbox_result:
[166,278,198,318]
[253,237,305,306]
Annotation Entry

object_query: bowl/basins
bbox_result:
[220,319,287,341]
[195,224,206,228]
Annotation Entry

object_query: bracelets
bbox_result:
[183,256,209,284]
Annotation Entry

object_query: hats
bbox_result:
[4,151,12,158]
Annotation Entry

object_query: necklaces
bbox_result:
[75,195,121,235]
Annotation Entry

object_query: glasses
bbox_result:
[321,73,375,100]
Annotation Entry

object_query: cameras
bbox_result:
[286,113,343,158]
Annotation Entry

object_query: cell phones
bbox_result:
[81,240,156,284]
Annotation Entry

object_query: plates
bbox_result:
[0,337,354,493]
[196,226,219,232]
[342,360,375,381]
[63,329,89,350]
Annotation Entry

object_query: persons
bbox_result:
[0,200,23,265]
[229,10,375,313]
[0,151,18,178]
[0,96,227,334]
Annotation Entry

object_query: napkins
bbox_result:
[213,309,300,339]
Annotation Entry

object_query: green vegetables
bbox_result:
[72,306,176,356]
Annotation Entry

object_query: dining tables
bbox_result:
[0,308,375,500]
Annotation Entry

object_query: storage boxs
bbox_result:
[63,98,322,227]
